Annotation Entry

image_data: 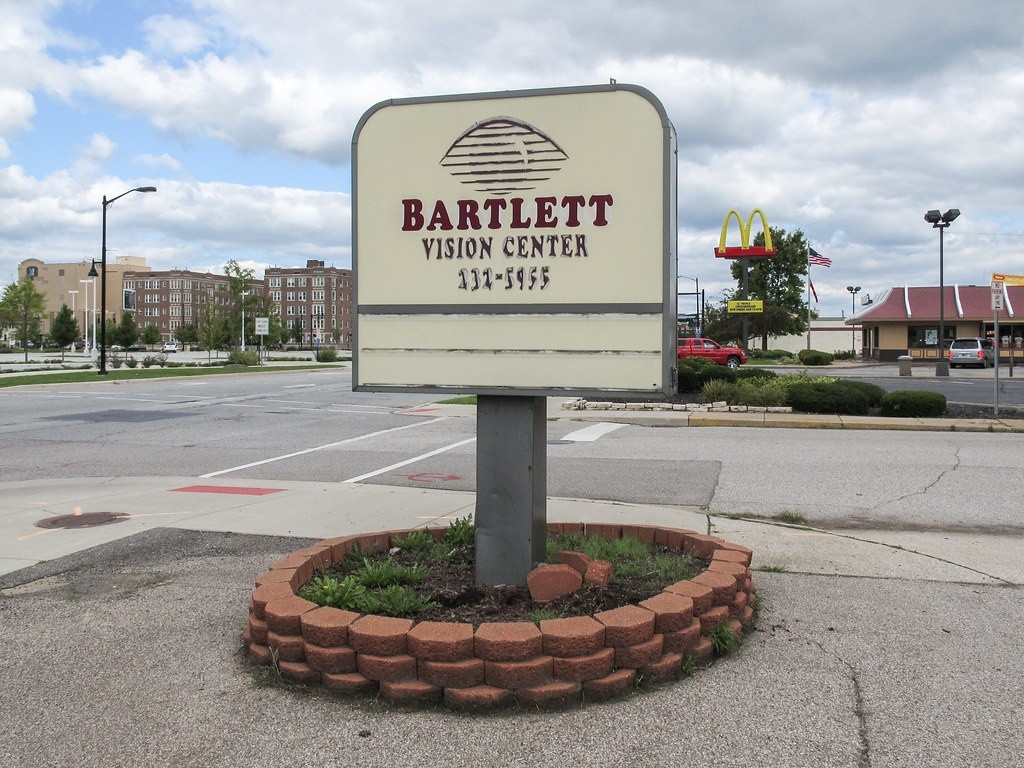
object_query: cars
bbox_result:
[28,340,34,347]
[160,341,178,353]
[65,342,147,351]
[948,337,996,368]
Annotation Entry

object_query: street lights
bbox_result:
[96,186,158,374]
[677,274,700,329]
[68,290,79,353]
[846,286,862,351]
[80,279,93,354]
[923,208,961,363]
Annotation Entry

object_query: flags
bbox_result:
[810,279,818,303]
[809,246,832,268]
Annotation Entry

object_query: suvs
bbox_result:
[677,337,748,371]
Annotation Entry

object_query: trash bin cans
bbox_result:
[897,356,914,376]
